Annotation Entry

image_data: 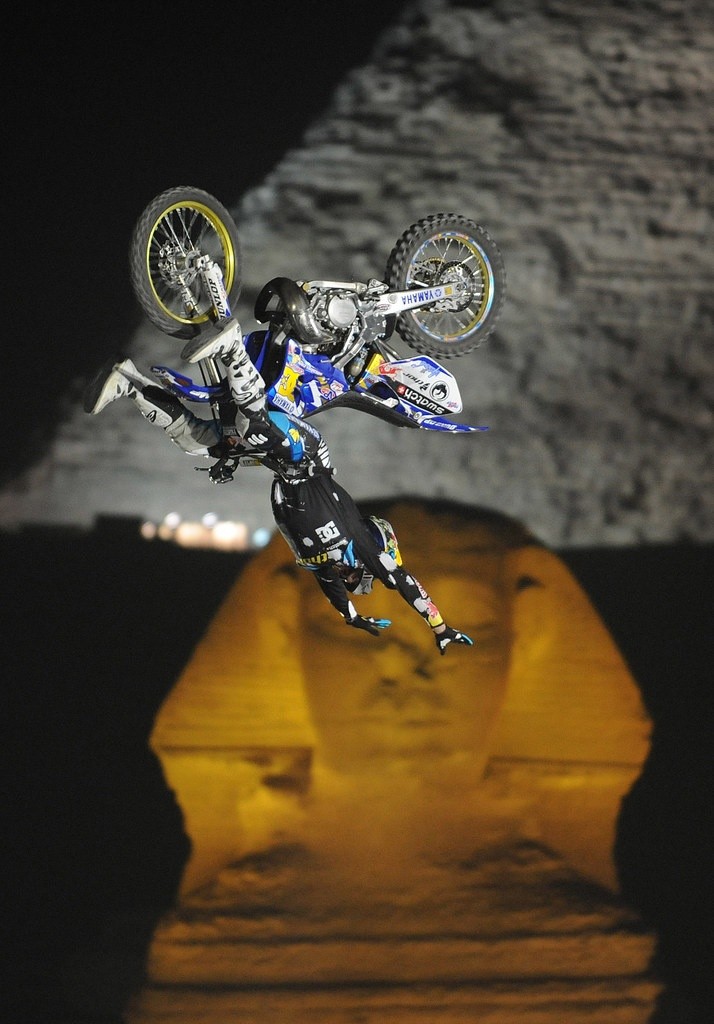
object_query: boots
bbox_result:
[84,352,184,428]
[180,316,267,406]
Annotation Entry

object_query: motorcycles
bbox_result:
[130,181,496,486]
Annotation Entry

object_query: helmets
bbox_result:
[342,515,402,597]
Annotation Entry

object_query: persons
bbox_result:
[85,314,478,660]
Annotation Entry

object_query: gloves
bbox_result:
[346,614,392,637]
[434,625,474,656]
